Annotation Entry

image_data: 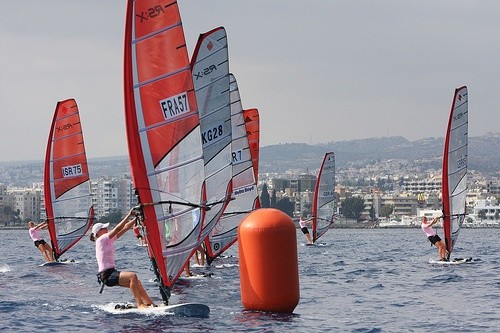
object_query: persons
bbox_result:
[421,216,447,261]
[184,259,191,278]
[299,216,313,244]
[192,244,205,267]
[133,223,146,246]
[28,220,56,263]
[88,209,158,309]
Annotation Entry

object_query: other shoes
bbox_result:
[437,258,442,261]
[442,258,448,261]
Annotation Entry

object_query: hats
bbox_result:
[92,222,110,236]
